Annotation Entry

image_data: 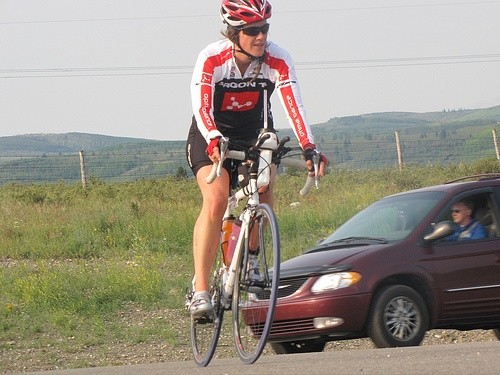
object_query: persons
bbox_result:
[188,0,331,321]
[435,199,486,240]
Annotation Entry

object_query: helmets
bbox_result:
[220,0,271,26]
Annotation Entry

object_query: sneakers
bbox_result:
[248,257,264,282]
[188,294,212,319]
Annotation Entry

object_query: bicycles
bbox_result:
[188,130,322,367]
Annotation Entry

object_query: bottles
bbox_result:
[225,214,243,262]
[221,214,237,265]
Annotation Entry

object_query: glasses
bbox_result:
[450,209,467,213]
[235,23,269,36]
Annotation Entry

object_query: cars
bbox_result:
[240,172,500,356]
